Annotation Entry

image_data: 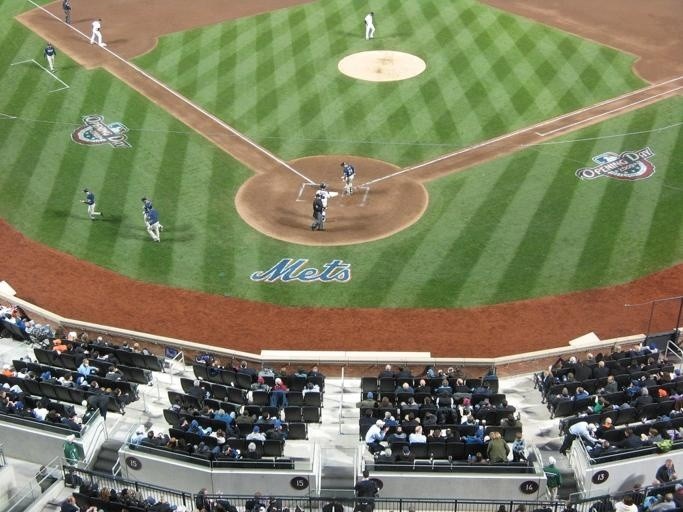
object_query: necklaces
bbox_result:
[340,161,355,196]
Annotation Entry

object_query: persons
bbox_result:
[310,193,325,232]
[89,19,107,46]
[144,208,160,242]
[79,189,103,221]
[140,197,164,232]
[42,43,56,71]
[62,0,70,24]
[364,12,375,41]
[2,302,683,512]
[314,182,330,229]
